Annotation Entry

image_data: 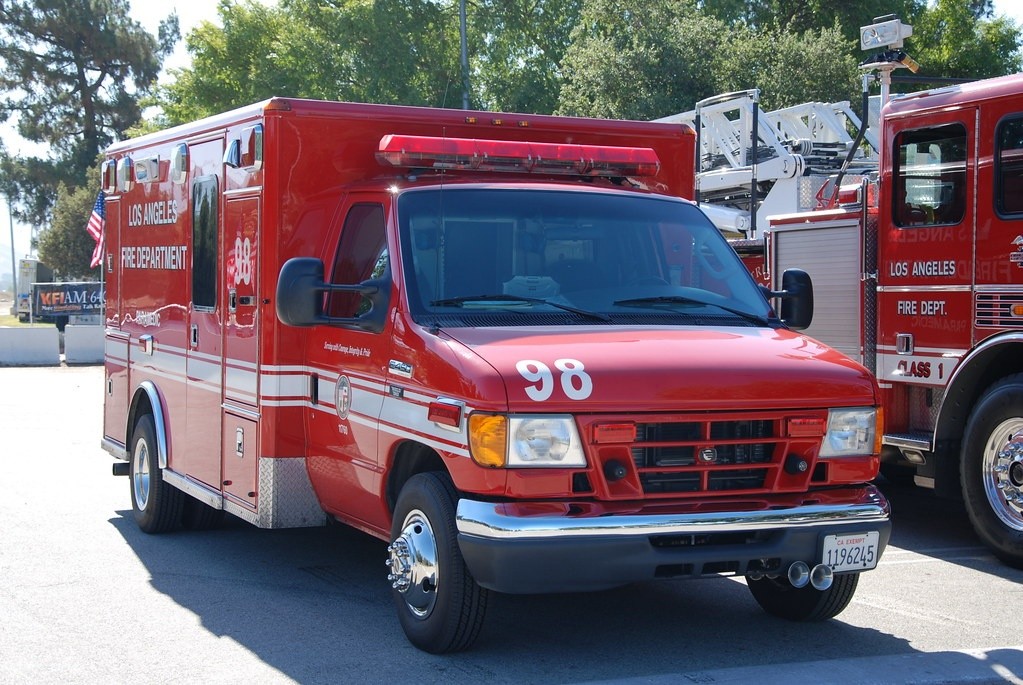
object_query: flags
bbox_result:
[86,190,106,270]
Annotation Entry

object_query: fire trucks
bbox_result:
[645,11,1023,572]
[99,95,892,657]
